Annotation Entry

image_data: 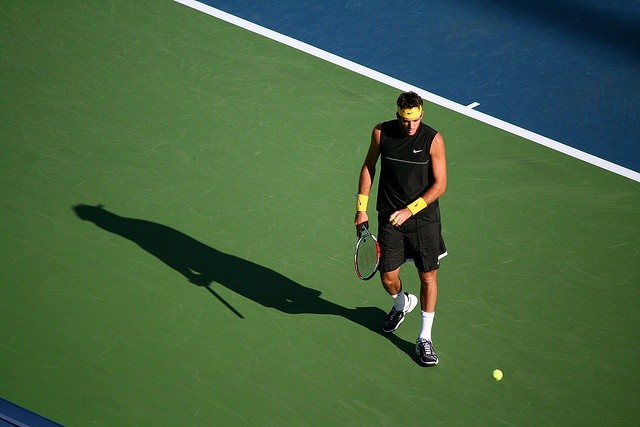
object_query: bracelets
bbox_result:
[356,195,368,212]
[407,196,428,216]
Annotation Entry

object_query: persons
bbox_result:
[354,91,449,367]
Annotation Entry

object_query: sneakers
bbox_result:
[384,292,418,333]
[416,337,439,366]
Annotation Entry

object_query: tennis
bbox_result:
[492,369,504,381]
